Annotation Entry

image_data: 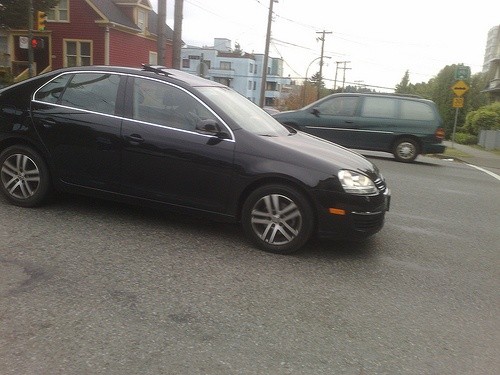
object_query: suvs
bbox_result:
[273,92,447,163]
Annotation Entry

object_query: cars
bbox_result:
[264,108,281,116]
[1,60,393,256]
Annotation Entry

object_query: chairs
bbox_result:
[162,90,180,123]
[136,88,152,122]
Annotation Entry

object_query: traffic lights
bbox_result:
[29,7,49,52]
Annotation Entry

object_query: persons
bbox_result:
[0,51,12,84]
